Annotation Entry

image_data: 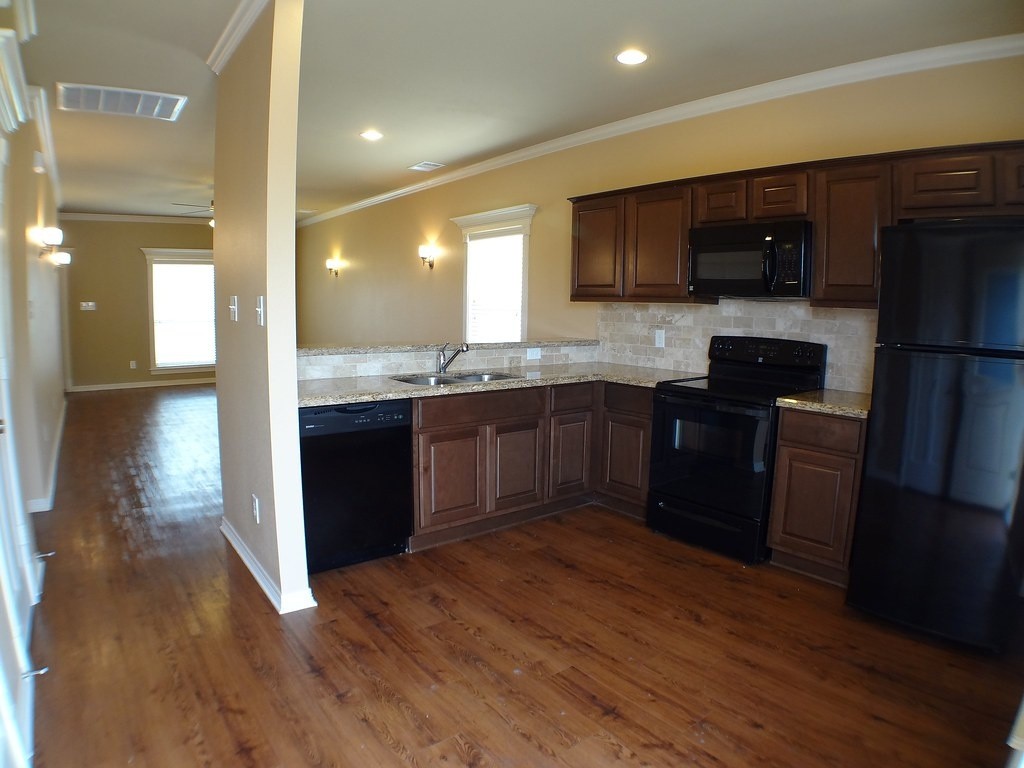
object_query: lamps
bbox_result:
[40,229,74,266]
[419,241,442,268]
[325,259,341,278]
[209,218,215,228]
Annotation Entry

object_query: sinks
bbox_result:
[395,376,463,385]
[453,374,518,381]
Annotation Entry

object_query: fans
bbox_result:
[171,200,214,214]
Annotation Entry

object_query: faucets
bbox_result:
[436,341,469,374]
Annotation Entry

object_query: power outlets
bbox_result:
[655,330,665,347]
[251,494,259,524]
[526,347,542,359]
[129,361,136,369]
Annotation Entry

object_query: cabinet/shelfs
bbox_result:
[407,381,654,554]
[767,408,867,588]
[567,140,1024,308]
